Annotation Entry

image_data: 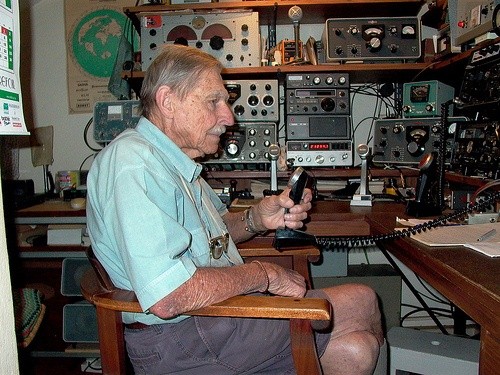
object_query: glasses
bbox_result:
[210,232,229,260]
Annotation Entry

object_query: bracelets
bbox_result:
[251,260,269,293]
[240,206,267,235]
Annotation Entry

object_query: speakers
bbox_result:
[61,258,99,348]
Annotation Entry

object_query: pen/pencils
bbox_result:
[477,228,497,241]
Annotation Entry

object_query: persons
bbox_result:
[85,44,387,375]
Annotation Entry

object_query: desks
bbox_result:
[12,198,500,375]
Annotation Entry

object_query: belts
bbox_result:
[123,323,149,329]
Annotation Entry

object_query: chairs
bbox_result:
[80,241,333,375]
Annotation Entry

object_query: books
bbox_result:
[395,222,500,247]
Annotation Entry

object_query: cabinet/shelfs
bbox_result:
[120,0,500,197]
